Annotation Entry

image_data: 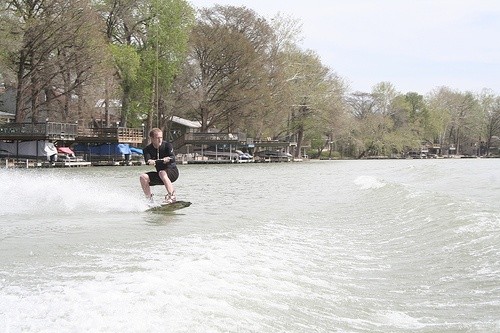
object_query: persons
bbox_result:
[139,127,180,207]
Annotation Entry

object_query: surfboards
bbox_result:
[144,201,192,212]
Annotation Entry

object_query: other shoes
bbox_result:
[161,193,176,205]
